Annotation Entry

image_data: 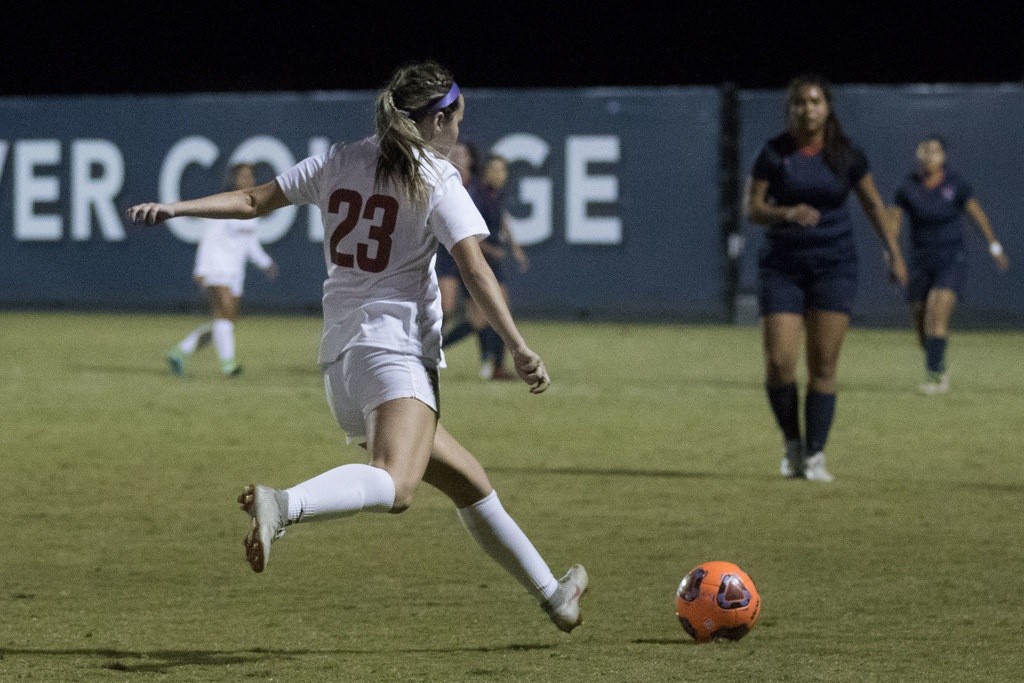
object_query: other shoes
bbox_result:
[915,371,949,397]
[219,364,245,381]
[477,364,519,381]
[168,348,188,379]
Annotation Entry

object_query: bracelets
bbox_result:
[988,241,1003,257]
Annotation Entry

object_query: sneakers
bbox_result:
[780,441,803,478]
[237,482,287,574]
[547,564,590,635]
[803,451,835,484]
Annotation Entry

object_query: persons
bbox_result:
[883,135,1008,395]
[742,76,907,483]
[171,164,278,379]
[435,141,528,382]
[126,64,589,635]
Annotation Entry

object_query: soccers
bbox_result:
[672,557,763,643]
